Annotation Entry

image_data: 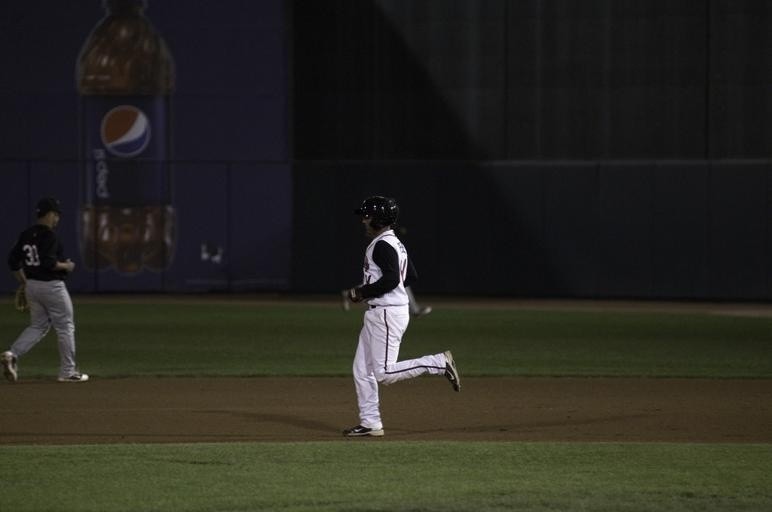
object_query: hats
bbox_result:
[34,197,66,216]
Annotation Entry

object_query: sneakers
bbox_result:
[443,352,461,391]
[59,370,90,383]
[343,425,385,438]
[2,349,24,382]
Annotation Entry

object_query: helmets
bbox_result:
[361,195,398,224]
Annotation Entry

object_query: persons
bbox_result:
[341,194,464,439]
[0,196,91,382]
[341,215,434,321]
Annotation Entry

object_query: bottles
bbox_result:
[79,1,175,276]
